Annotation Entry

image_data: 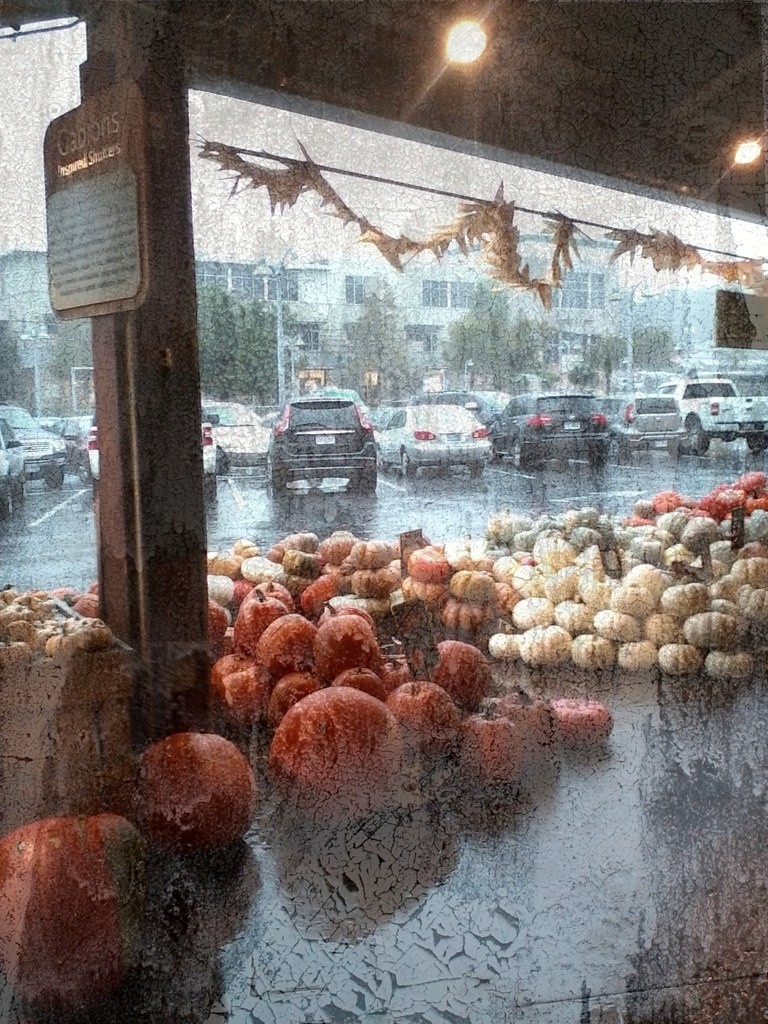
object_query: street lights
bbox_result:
[283,334,305,397]
[21,330,50,416]
[463,357,474,388]
[611,280,653,394]
[252,256,289,408]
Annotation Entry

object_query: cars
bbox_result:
[0,389,617,515]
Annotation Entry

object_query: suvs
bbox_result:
[596,394,692,459]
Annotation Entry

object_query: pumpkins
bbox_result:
[0,583,120,667]
[0,811,149,1024]
[133,728,256,855]
[207,470,768,832]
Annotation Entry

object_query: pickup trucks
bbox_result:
[657,377,767,455]
